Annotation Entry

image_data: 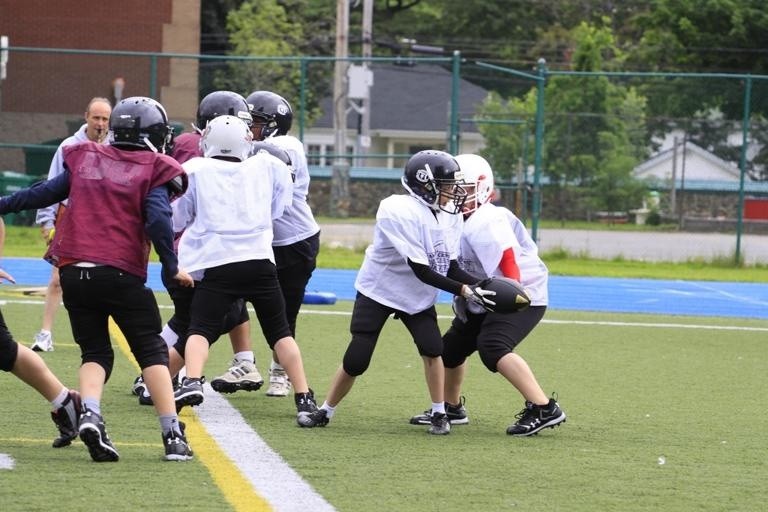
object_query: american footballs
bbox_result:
[479,278,530,314]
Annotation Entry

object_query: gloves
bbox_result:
[461,278,499,312]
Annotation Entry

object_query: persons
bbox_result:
[211,90,320,396]
[133,92,253,405]
[1,96,194,461]
[0,218,81,447]
[296,150,497,435]
[409,154,566,437]
[174,115,319,417]
[30,97,114,352]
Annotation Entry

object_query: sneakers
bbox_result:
[506,399,567,437]
[131,360,330,462]
[78,410,123,463]
[409,395,469,426]
[28,327,56,352]
[49,388,83,451]
[425,409,454,435]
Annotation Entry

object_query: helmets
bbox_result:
[197,89,254,140]
[398,149,470,215]
[103,95,174,152]
[244,89,294,139]
[198,113,256,162]
[448,151,496,214]
